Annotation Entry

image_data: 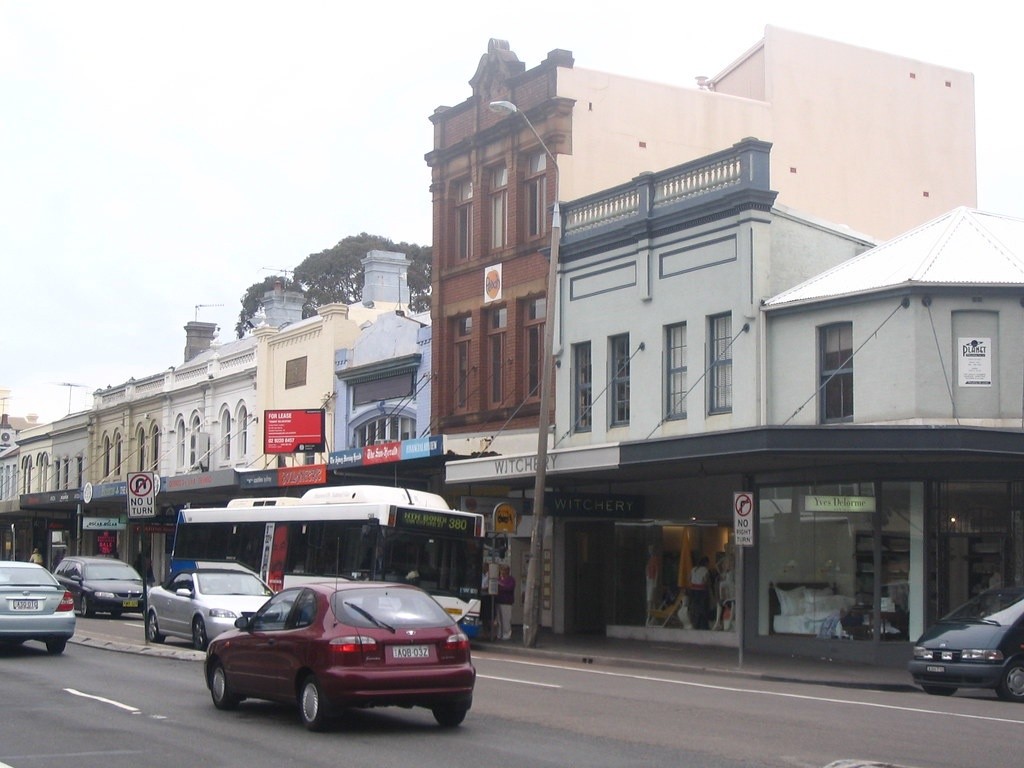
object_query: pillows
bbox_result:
[801,588,834,612]
[774,586,806,617]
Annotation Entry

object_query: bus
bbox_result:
[169,480,507,644]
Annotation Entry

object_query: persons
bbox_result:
[29,547,44,565]
[646,544,661,609]
[689,553,712,630]
[479,554,515,641]
[713,556,735,631]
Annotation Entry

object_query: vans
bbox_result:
[908,581,1024,704]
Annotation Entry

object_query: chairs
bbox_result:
[644,588,685,627]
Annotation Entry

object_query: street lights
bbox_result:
[487,100,562,646]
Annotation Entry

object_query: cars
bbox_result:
[144,567,291,651]
[204,579,477,733]
[0,560,76,653]
[53,556,147,619]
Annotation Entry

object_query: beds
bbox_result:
[767,580,901,639]
